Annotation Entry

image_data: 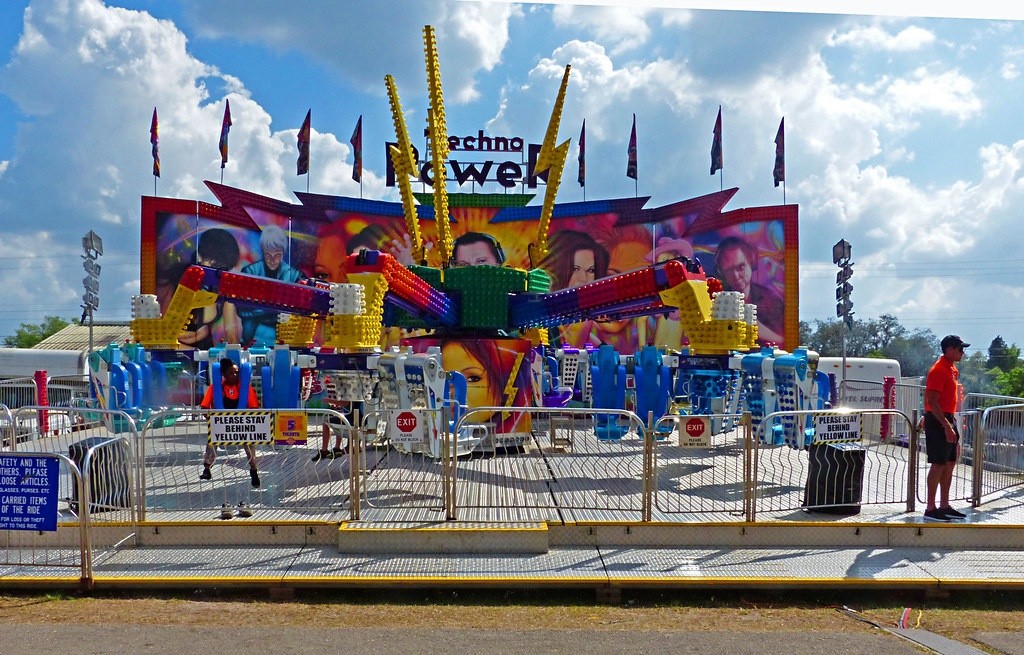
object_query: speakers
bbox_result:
[69,437,131,514]
[801,443,866,515]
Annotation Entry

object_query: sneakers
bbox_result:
[923,508,951,522]
[221,502,232,519]
[238,501,252,516]
[939,505,967,518]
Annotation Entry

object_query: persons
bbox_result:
[311,399,368,461]
[72,416,89,433]
[924,335,970,521]
[199,358,261,489]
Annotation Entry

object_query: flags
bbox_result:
[774,117,784,187]
[350,115,362,182]
[149,107,160,178]
[578,119,585,187]
[219,99,232,169]
[627,113,637,180]
[296,108,310,175]
[711,105,722,175]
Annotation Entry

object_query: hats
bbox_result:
[644,237,693,262]
[941,335,970,351]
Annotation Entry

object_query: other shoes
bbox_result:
[199,468,211,479]
[311,449,330,461]
[250,468,260,488]
[330,447,345,459]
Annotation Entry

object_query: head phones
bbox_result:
[714,236,758,280]
[450,233,506,267]
